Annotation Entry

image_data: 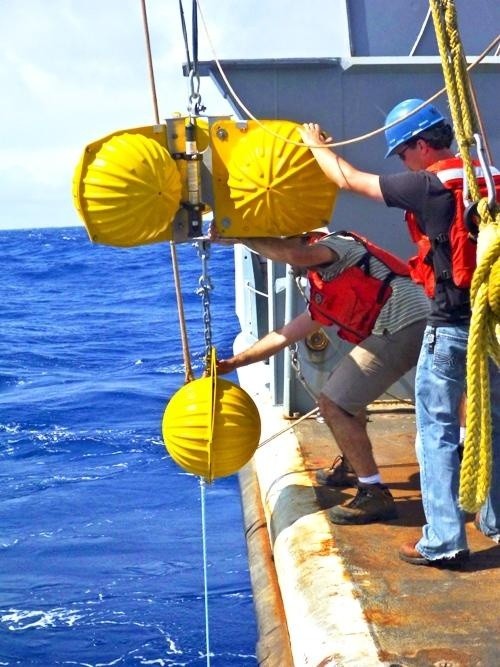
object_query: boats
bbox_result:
[137,0,500,666]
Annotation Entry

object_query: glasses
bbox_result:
[398,140,420,160]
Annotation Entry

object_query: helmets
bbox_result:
[384,99,445,159]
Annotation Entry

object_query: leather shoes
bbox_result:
[399,541,430,565]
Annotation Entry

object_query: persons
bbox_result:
[295,99,500,567]
[203,218,432,524]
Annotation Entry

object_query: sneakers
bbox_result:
[328,483,397,524]
[316,455,358,486]
